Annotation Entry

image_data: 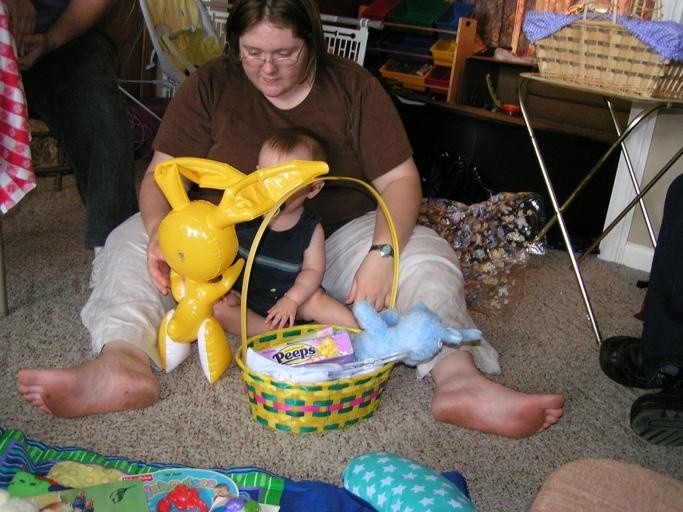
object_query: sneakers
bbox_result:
[599,336,675,390]
[631,380,682,448]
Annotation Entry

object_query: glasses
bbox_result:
[234,42,305,66]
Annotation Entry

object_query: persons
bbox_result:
[0,0,139,258]
[212,128,360,338]
[209,482,235,502]
[16,0,565,440]
[599,174,683,447]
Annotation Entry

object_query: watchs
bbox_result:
[369,244,395,258]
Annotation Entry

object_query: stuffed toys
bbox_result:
[350,296,481,368]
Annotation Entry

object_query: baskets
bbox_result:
[527,1,683,104]
[237,177,400,434]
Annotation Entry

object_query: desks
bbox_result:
[518,72,683,347]
